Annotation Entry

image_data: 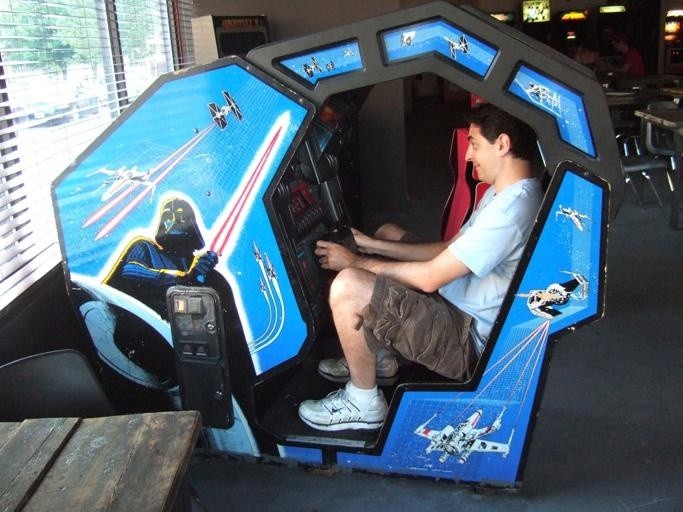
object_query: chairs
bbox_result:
[0,345,121,422]
[610,99,680,226]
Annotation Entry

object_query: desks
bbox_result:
[1,410,204,511]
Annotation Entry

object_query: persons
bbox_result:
[562,23,645,88]
[99,190,281,458]
[298,102,546,432]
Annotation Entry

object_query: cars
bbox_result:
[12,75,90,126]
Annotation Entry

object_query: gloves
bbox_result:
[158,251,219,285]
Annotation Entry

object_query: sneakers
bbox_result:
[299,388,389,431]
[318,352,399,386]
